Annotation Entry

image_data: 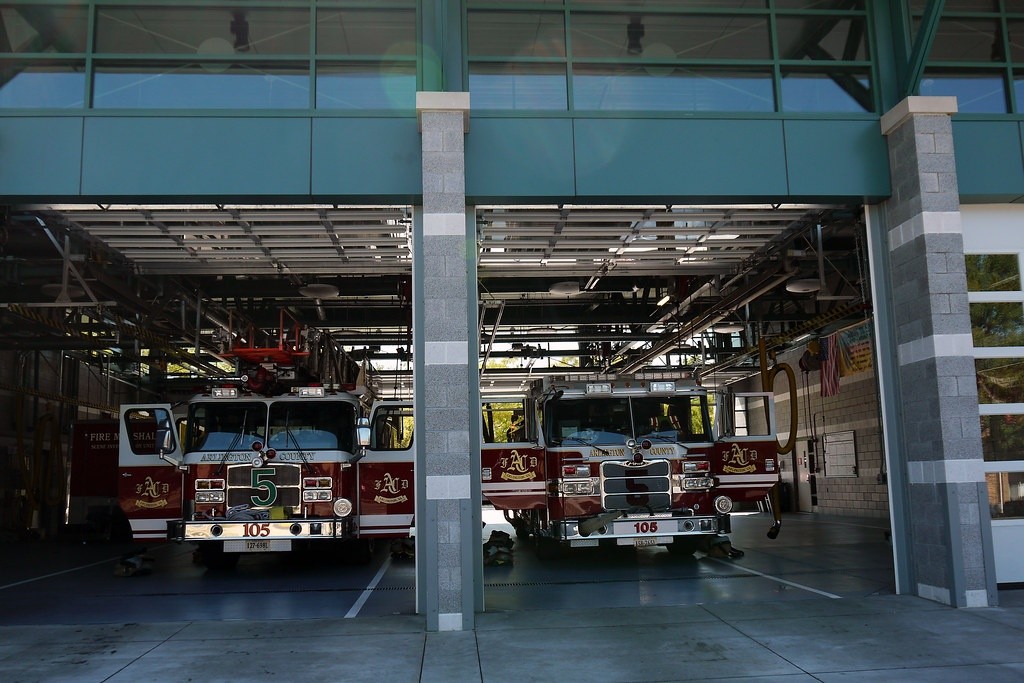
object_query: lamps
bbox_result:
[41,271,86,299]
[713,324,744,333]
[547,281,588,296]
[786,277,820,292]
[300,284,340,299]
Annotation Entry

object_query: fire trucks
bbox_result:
[116,305,415,568]
[484,371,778,561]
